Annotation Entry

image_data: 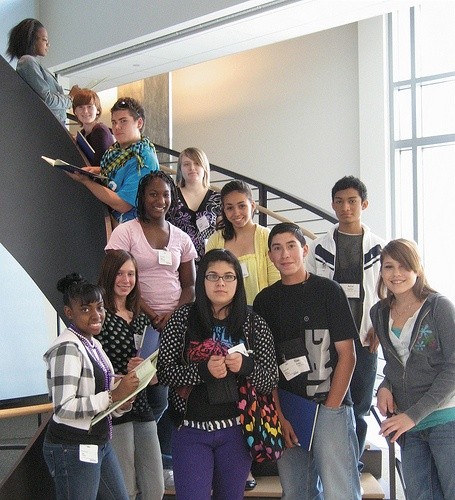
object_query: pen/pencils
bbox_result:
[109,374,125,377]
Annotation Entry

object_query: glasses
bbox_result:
[113,101,139,117]
[204,273,236,282]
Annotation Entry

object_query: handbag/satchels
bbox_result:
[239,313,285,463]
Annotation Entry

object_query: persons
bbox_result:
[72,88,115,186]
[155,248,280,499]
[206,179,283,491]
[63,97,160,230]
[95,250,165,500]
[103,169,199,470]
[252,222,365,499]
[42,273,140,500]
[164,147,225,303]
[6,18,82,127]
[300,175,392,481]
[369,238,455,500]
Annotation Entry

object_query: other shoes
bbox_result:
[163,469,175,489]
[244,480,256,490]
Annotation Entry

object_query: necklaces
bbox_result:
[68,327,94,348]
[395,299,418,318]
